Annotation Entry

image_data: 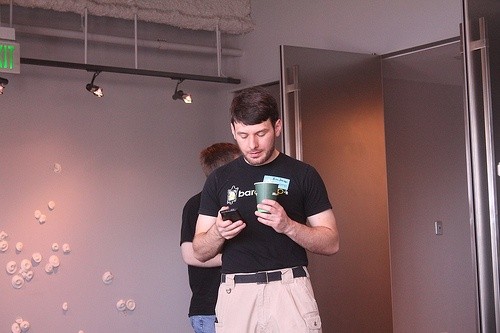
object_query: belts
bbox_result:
[219,267,307,284]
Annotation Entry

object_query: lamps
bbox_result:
[172,80,192,103]
[0,76,8,95]
[86,71,105,98]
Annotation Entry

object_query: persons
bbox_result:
[179,142,244,333]
[192,85,340,333]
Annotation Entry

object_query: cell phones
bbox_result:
[221,209,247,227]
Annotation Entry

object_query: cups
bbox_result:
[254,182,279,214]
